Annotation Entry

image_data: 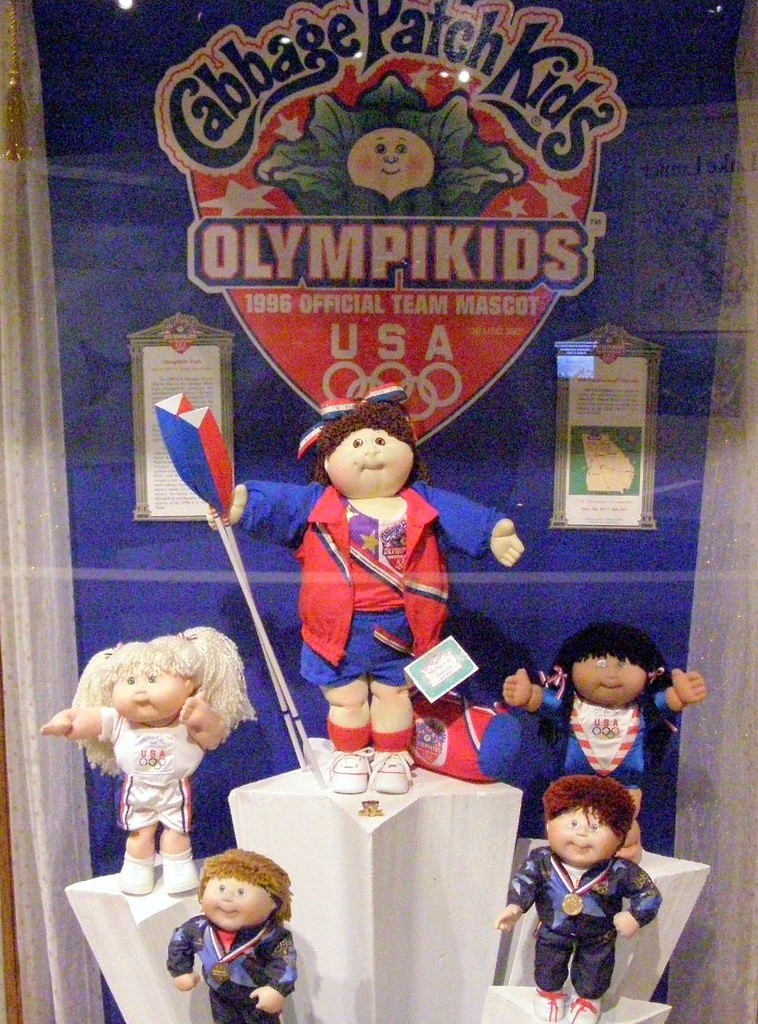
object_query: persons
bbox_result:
[204,381,524,793]
[502,623,708,865]
[39,625,258,895]
[495,774,664,1024]
[167,848,298,1024]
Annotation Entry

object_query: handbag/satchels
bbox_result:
[405,690,500,784]
